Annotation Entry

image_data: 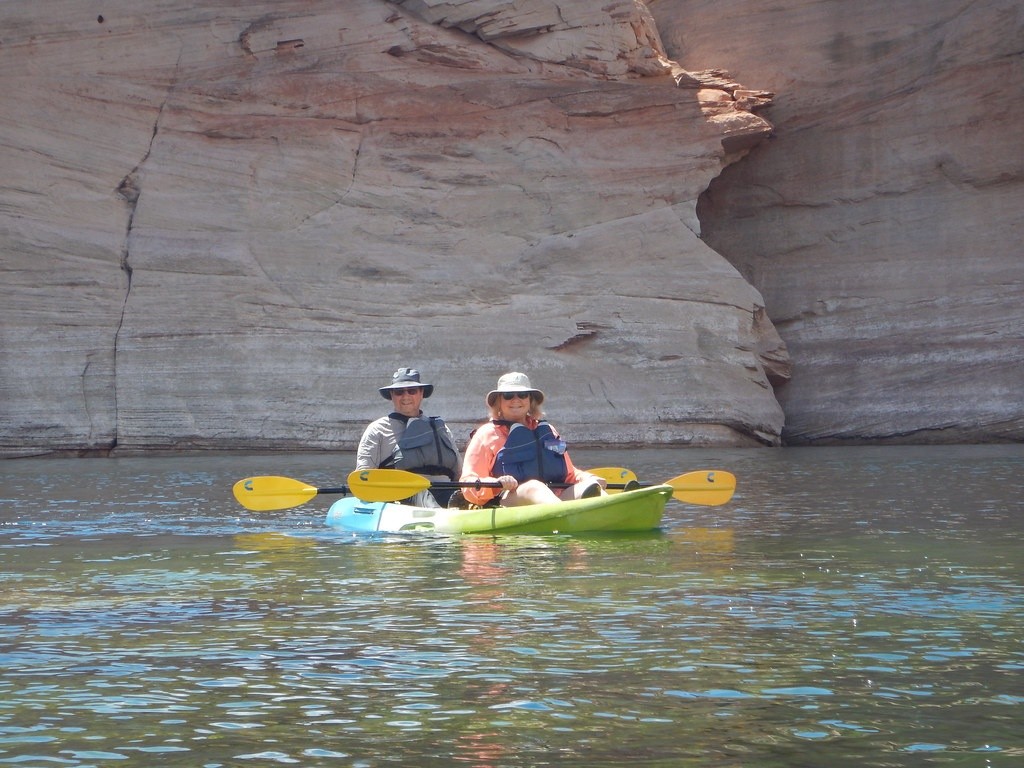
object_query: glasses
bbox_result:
[503,392,528,400]
[393,388,418,395]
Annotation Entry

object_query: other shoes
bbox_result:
[448,490,469,510]
[580,483,601,498]
[624,480,639,491]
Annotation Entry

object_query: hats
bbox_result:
[486,372,545,408]
[378,367,434,400]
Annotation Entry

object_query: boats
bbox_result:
[325,487,674,538]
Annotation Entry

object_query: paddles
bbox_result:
[347,468,737,506]
[233,468,638,511]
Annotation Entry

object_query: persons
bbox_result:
[460,372,640,508]
[355,368,474,510]
